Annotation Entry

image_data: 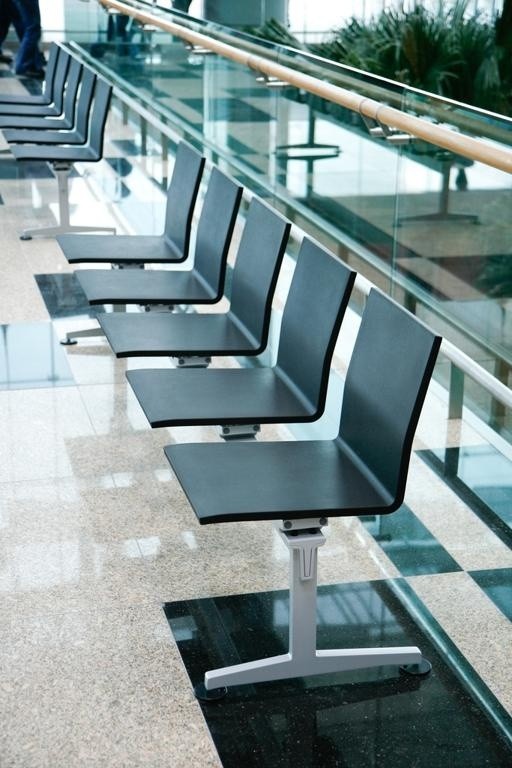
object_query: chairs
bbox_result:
[94,192,293,372]
[0,37,128,240]
[272,74,484,232]
[52,139,207,273]
[71,165,246,313]
[160,282,445,707]
[127,230,360,445]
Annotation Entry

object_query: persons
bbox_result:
[171,0,191,41]
[12,0,45,80]
[0,0,47,66]
[107,0,129,43]
